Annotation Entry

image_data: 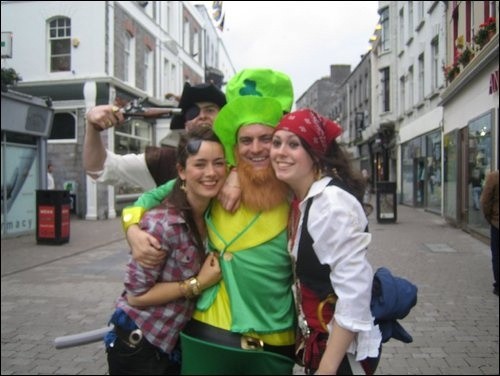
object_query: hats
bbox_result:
[213,67,294,166]
[178,81,227,109]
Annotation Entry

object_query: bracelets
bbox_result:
[225,180,242,189]
[189,277,200,296]
[179,280,192,299]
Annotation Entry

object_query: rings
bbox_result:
[106,120,113,128]
[112,105,119,113]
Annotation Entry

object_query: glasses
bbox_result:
[186,131,215,154]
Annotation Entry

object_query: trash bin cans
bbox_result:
[376,182,397,223]
[35,188,71,246]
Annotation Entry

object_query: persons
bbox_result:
[82,82,227,193]
[269,109,418,376]
[47,165,56,190]
[122,68,301,376]
[480,164,500,296]
[105,127,226,376]
[360,169,372,211]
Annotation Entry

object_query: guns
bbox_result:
[93,95,182,131]
